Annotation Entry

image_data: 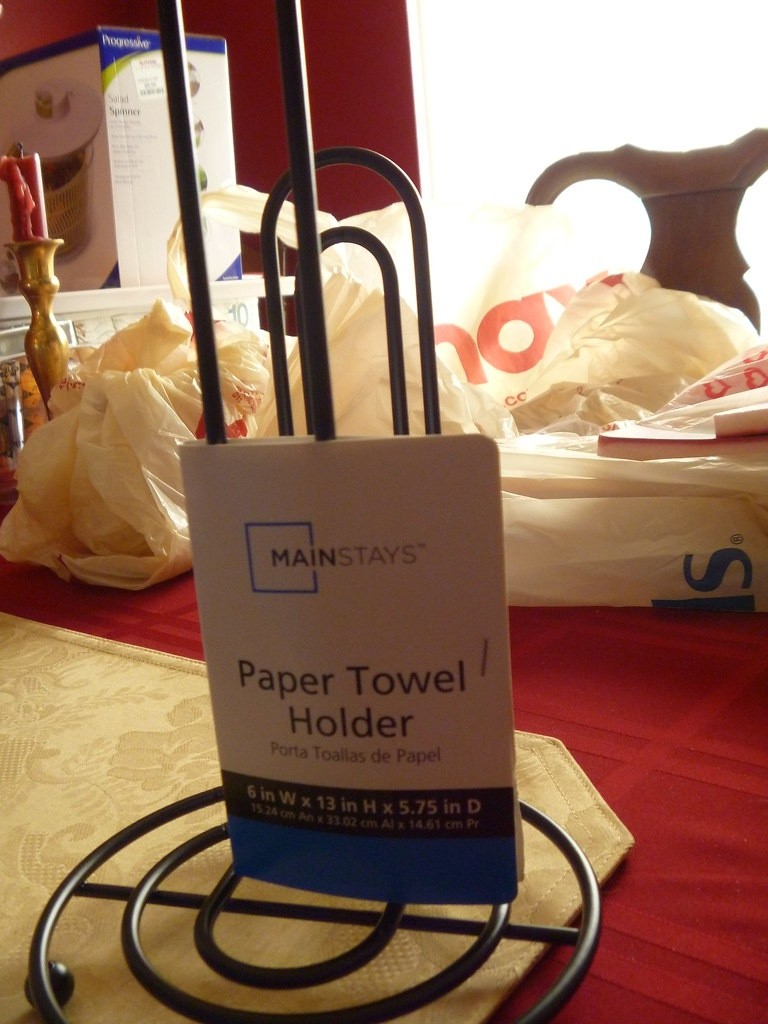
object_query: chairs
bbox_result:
[524,127,768,335]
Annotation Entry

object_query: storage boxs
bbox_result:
[0,274,296,350]
[0,26,243,299]
